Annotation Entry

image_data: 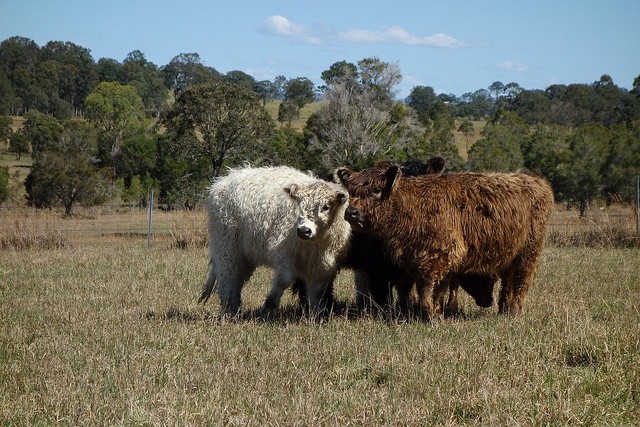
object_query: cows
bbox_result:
[334,159,556,325]
[196,160,355,324]
